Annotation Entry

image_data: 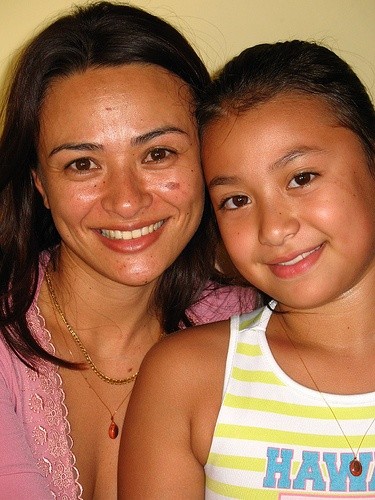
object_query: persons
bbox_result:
[116,38,375,500]
[0,1,214,500]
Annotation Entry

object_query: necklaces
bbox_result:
[44,268,138,440]
[276,314,375,477]
